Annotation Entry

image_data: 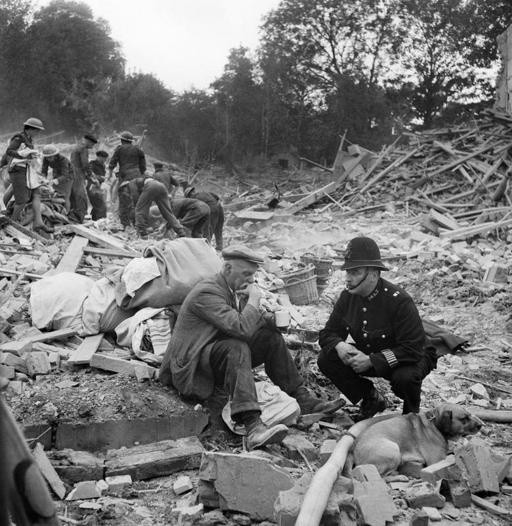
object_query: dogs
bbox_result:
[342,404,483,483]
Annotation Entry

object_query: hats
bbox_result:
[222,243,264,264]
[340,236,390,272]
[23,117,45,130]
[153,162,163,168]
[41,144,60,158]
[116,180,130,191]
[118,130,135,142]
[96,150,109,158]
[84,134,98,144]
[185,186,195,197]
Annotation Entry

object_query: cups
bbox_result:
[275,310,291,328]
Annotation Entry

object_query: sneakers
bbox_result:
[140,232,148,240]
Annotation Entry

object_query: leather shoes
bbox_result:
[34,224,56,234]
[349,392,387,424]
[247,423,288,451]
[402,401,421,414]
[300,398,346,417]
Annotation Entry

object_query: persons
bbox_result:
[38,133,226,250]
[5,117,56,234]
[162,244,346,453]
[316,236,429,414]
